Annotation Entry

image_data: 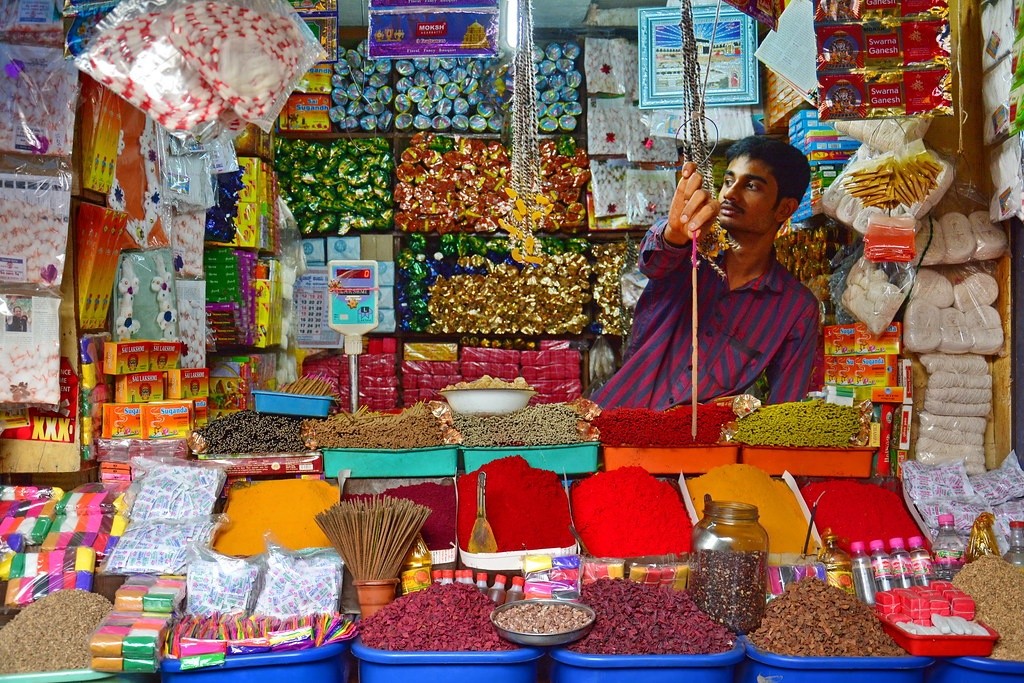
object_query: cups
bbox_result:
[352,578,398,619]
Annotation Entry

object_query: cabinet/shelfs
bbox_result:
[279,27,649,409]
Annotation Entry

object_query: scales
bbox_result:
[327,261,379,411]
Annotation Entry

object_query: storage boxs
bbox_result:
[0,64,1024,683]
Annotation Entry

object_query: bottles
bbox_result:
[930,514,967,583]
[1003,520,1024,568]
[431,568,525,608]
[851,536,935,606]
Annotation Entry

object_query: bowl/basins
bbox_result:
[434,389,538,416]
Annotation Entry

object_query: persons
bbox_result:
[588,136,819,413]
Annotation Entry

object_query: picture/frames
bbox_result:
[639,6,760,108]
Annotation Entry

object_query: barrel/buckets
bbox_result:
[687,500,768,635]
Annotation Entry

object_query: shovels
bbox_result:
[469,471,499,552]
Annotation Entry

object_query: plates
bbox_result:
[492,600,596,645]
